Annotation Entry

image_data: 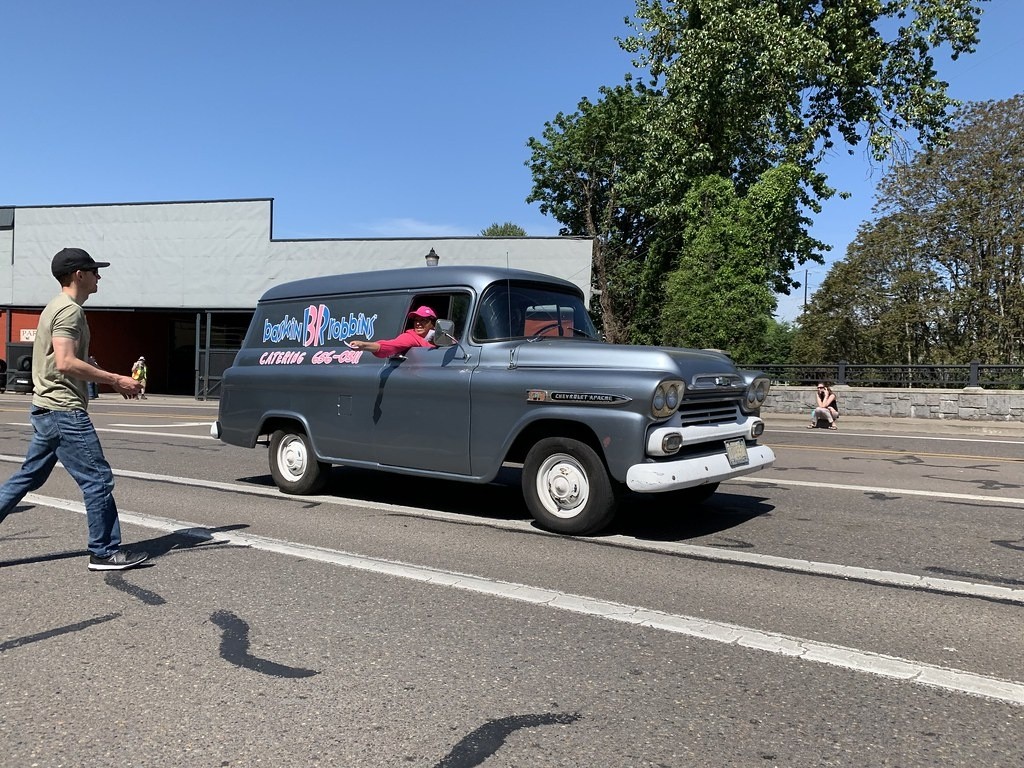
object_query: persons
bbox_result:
[348,306,443,359]
[131,356,147,400]
[86,355,99,398]
[0,247,150,572]
[807,383,839,430]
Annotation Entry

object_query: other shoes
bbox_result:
[806,422,818,428]
[830,426,839,430]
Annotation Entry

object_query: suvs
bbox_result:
[206,264,779,540]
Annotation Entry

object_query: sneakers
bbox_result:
[86,549,148,571]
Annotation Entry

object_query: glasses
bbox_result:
[817,386,825,389]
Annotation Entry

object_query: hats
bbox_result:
[51,248,110,276]
[407,304,436,319]
